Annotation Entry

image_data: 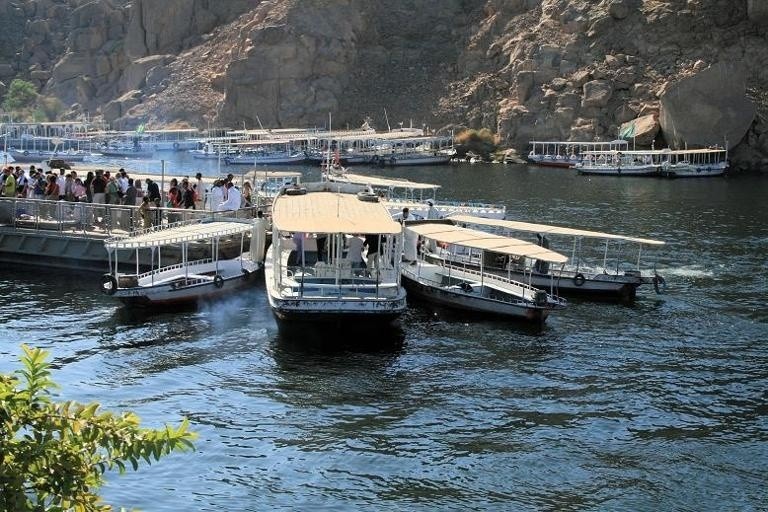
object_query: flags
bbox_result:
[620,125,634,140]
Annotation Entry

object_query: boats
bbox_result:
[527,138,730,177]
[15,213,77,229]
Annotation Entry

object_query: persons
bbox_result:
[391,208,415,224]
[0,165,252,212]
[293,234,387,274]
[427,199,439,219]
